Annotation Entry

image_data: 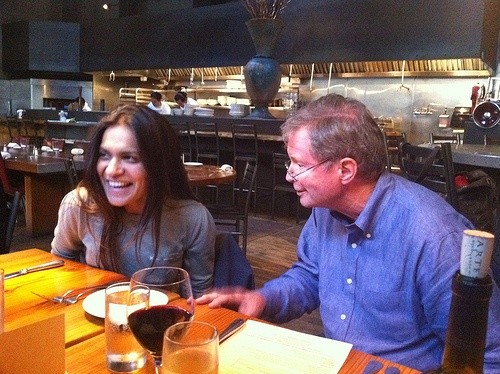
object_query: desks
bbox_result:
[419,137,500,172]
[184,165,237,202]
[0,248,179,348]
[0,145,86,238]
[52,296,429,374]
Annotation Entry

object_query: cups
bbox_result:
[105,282,150,374]
[159,321,219,374]
[20,137,26,148]
[52,138,59,151]
[0,268,5,344]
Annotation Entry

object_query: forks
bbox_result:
[30,281,120,304]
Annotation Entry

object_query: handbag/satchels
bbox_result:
[439,170,497,226]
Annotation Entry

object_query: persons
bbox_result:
[184,95,500,374]
[75,96,93,111]
[145,93,172,116]
[50,101,216,305]
[173,90,198,111]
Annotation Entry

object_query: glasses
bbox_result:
[285,157,330,182]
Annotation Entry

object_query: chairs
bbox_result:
[207,162,258,257]
[5,115,39,145]
[383,131,458,212]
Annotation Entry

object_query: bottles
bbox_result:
[3,144,7,152]
[62,104,68,121]
[420,230,496,374]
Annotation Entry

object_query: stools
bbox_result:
[178,120,305,223]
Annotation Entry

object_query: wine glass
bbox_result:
[125,266,195,374]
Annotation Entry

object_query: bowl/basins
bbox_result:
[267,107,290,118]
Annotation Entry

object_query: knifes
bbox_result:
[4,260,65,279]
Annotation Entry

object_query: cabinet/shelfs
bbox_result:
[118,87,298,116]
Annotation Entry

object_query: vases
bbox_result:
[239,17,285,120]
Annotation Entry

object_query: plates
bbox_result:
[13,146,30,149]
[171,103,250,117]
[82,286,169,319]
[47,148,63,153]
[196,95,252,106]
[184,162,203,166]
